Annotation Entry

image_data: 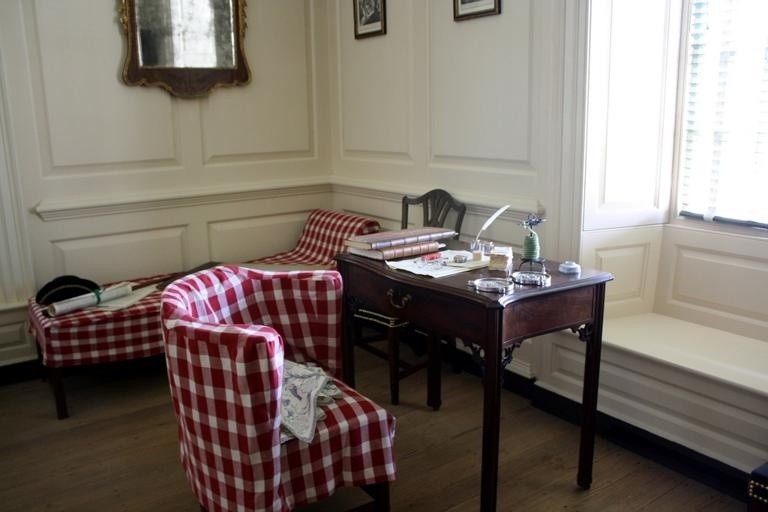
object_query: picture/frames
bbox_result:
[454,0,501,21]
[354,0,386,40]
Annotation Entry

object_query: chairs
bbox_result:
[355,189,466,412]
[159,263,397,512]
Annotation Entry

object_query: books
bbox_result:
[84,283,159,312]
[343,225,458,260]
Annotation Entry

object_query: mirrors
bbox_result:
[118,0,252,99]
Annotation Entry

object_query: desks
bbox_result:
[332,236,614,512]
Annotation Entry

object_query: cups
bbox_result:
[472,238,495,261]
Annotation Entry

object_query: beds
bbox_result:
[28,207,382,420]
